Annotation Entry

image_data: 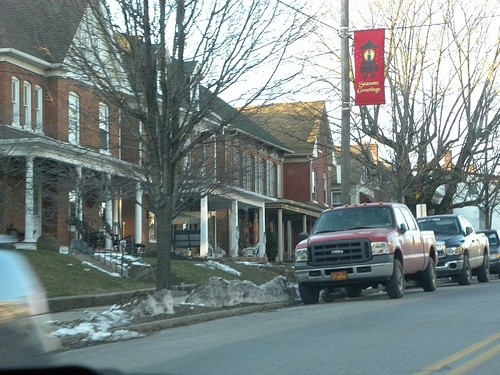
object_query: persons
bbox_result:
[5,223,24,242]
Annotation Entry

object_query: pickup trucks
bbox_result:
[292,201,438,305]
[416,214,500,286]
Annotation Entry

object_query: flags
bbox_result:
[354,29,386,105]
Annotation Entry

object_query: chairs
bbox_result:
[242,242,259,257]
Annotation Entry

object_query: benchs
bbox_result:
[174,230,212,257]
